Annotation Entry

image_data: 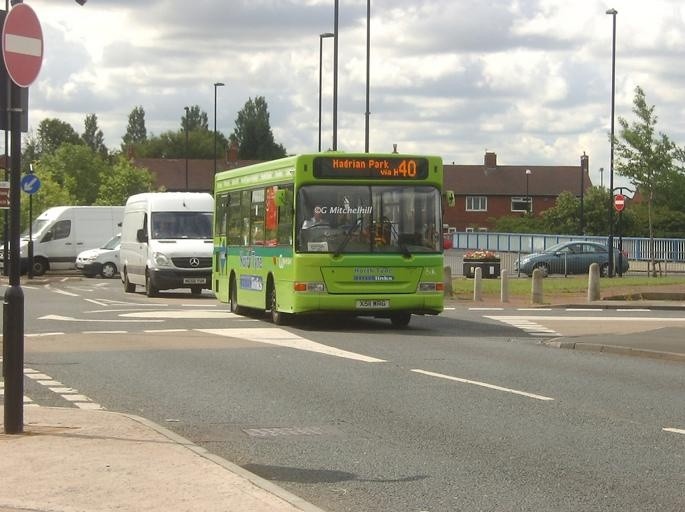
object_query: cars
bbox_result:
[514,241,629,278]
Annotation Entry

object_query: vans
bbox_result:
[0,192,214,298]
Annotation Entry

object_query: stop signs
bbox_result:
[1,2,44,87]
[614,194,625,211]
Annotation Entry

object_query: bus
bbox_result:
[212,153,455,326]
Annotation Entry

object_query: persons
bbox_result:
[301,204,331,230]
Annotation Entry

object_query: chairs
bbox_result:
[398,232,424,246]
[276,222,293,243]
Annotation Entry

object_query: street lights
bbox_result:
[526,169,531,213]
[319,33,335,153]
[605,8,618,278]
[214,82,225,175]
[184,107,189,192]
[599,168,604,189]
